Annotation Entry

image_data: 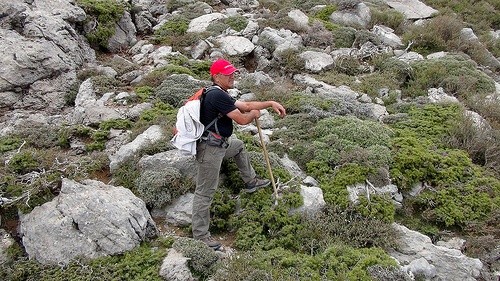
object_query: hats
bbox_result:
[209,58,240,76]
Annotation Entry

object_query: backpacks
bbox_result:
[169,84,231,156]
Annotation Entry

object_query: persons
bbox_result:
[191,59,287,250]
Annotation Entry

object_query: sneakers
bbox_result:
[244,178,271,193]
[206,241,221,250]
